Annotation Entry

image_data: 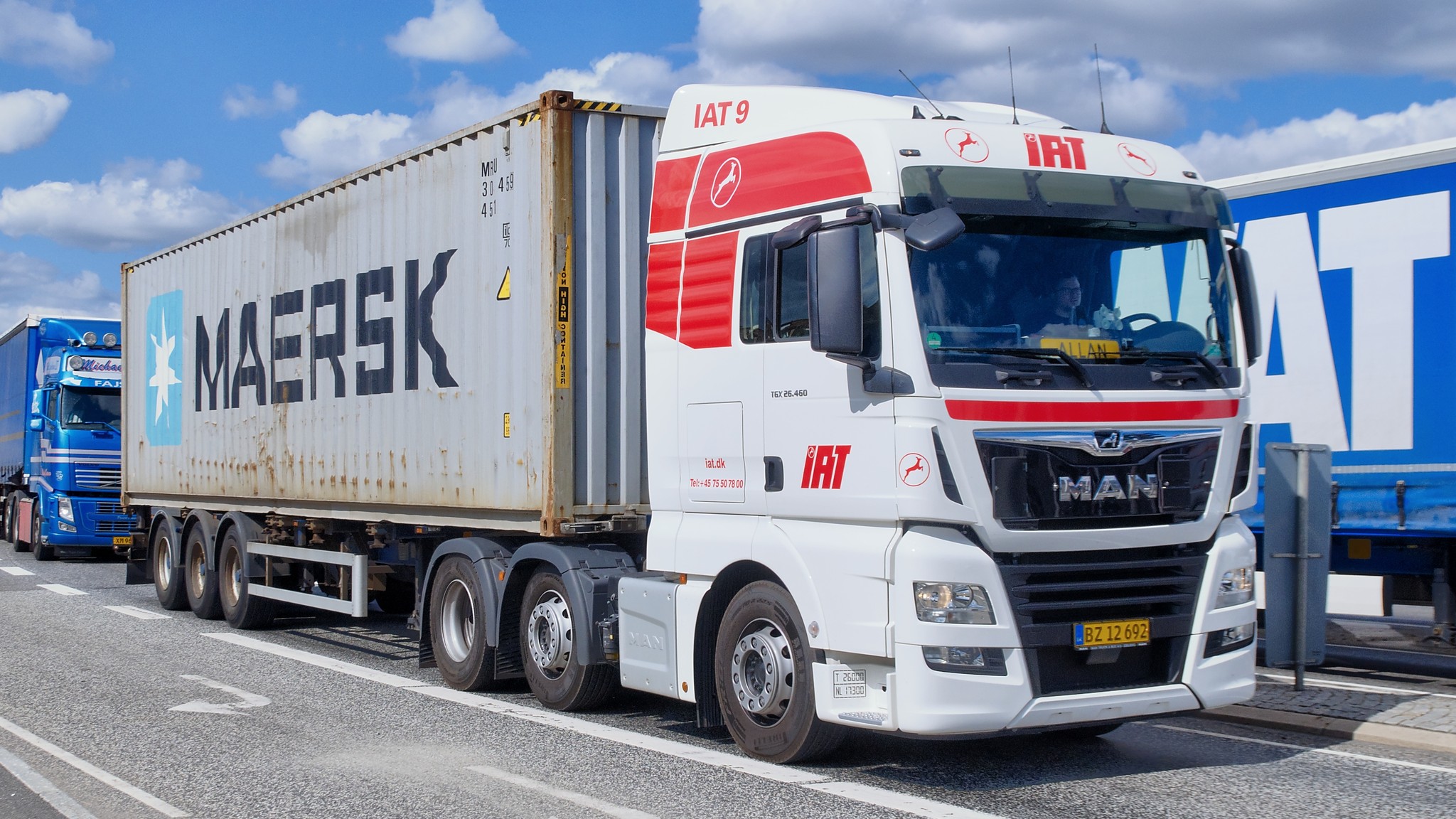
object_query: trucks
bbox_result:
[1109,135,1455,619]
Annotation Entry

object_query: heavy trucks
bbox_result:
[0,312,139,562]
[121,82,1266,768]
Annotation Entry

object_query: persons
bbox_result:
[1026,270,1082,334]
